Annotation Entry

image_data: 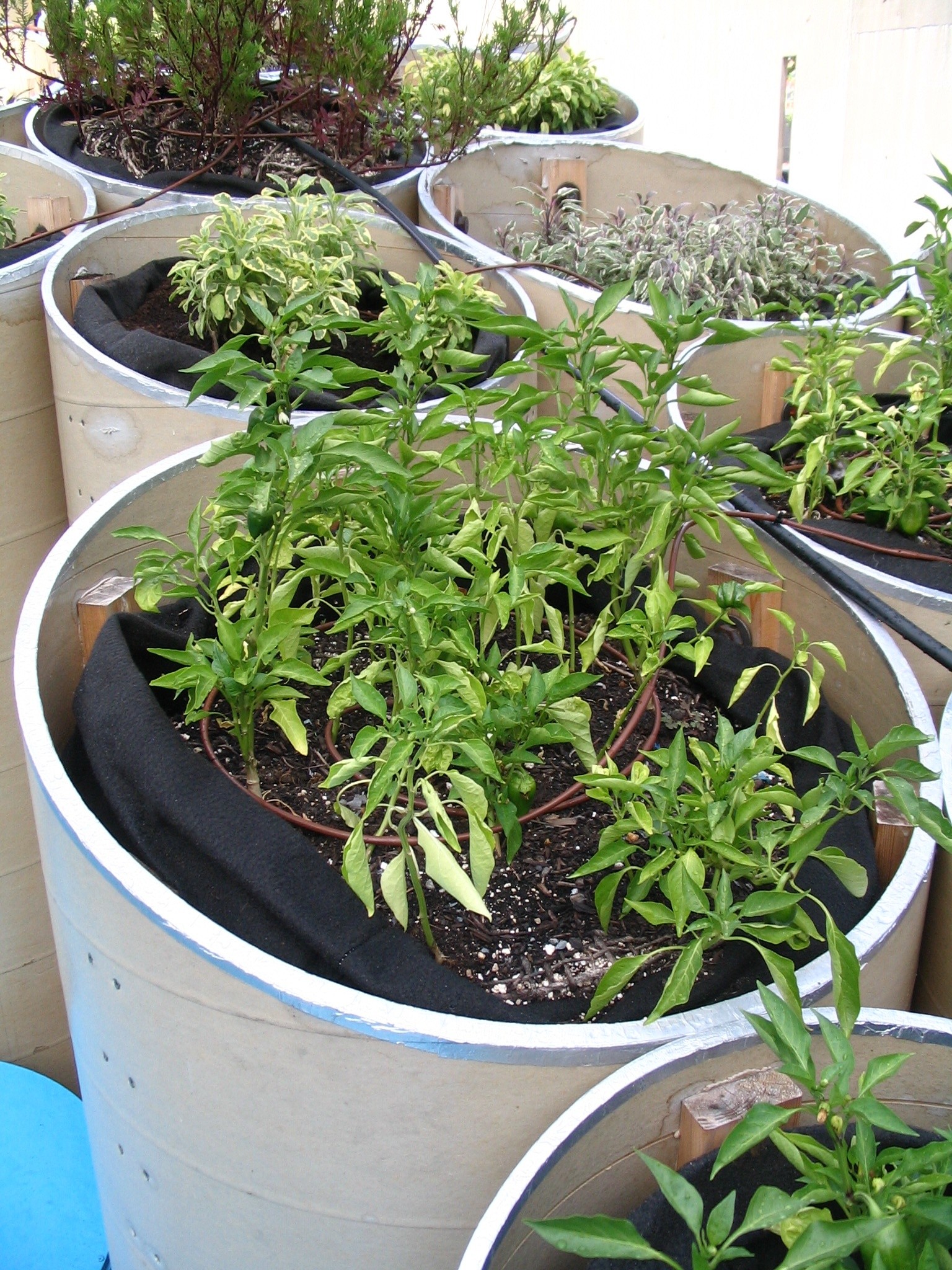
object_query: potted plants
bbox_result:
[1,0,952,1269]
